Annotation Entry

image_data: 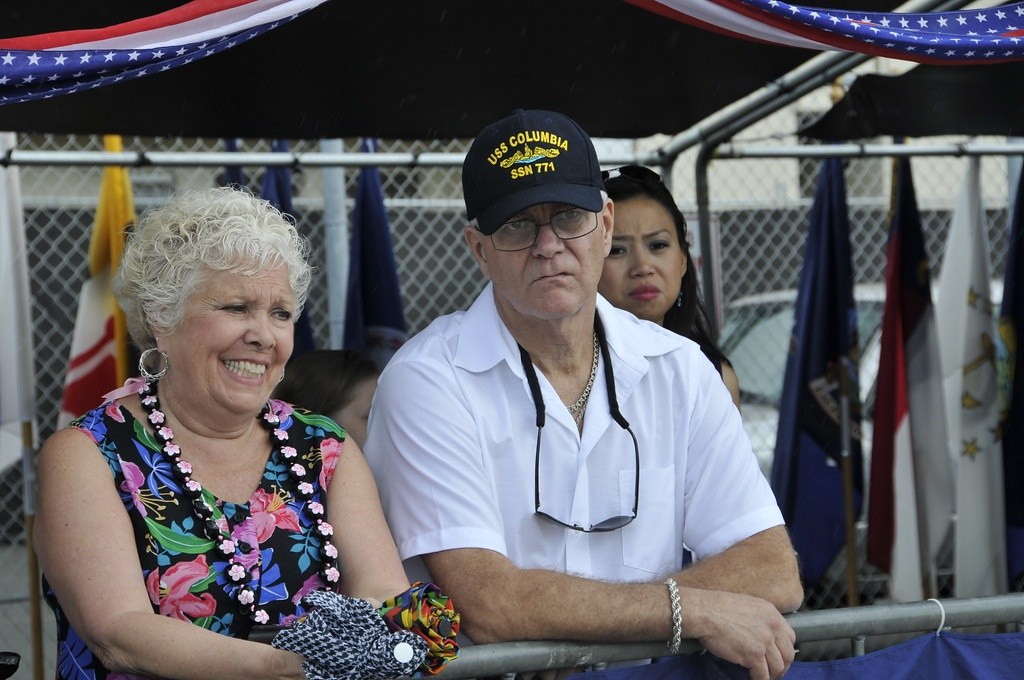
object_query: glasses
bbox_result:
[533,426,639,531]
[601,165,664,186]
[473,207,599,252]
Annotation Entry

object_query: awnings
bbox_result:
[2,0,1024,136]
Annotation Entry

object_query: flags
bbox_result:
[342,138,409,377]
[935,137,1023,599]
[0,133,39,471]
[769,154,865,613]
[867,137,954,600]
[221,140,314,358]
[58,134,133,428]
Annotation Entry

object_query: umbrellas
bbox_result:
[270,582,461,680]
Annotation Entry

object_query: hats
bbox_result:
[461,107,607,236]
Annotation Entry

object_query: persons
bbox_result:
[270,351,377,453]
[599,163,742,411]
[35,187,415,678]
[361,112,804,680]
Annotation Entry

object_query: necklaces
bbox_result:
[565,326,599,425]
[100,376,340,628]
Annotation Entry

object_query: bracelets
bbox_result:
[664,577,682,656]
